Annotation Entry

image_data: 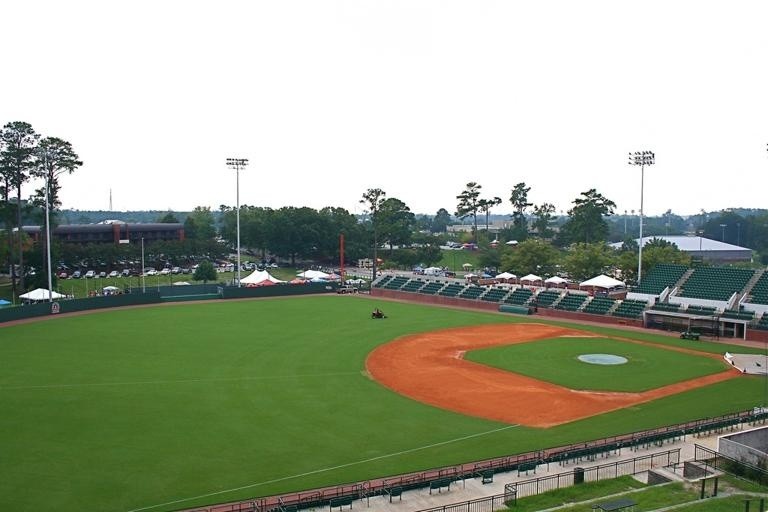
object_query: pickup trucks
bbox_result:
[336,284,357,294]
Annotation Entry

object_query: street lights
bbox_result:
[34,145,60,302]
[226,158,249,287]
[627,151,656,287]
[624,208,741,252]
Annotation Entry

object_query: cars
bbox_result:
[445,240,477,250]
[16,249,279,281]
